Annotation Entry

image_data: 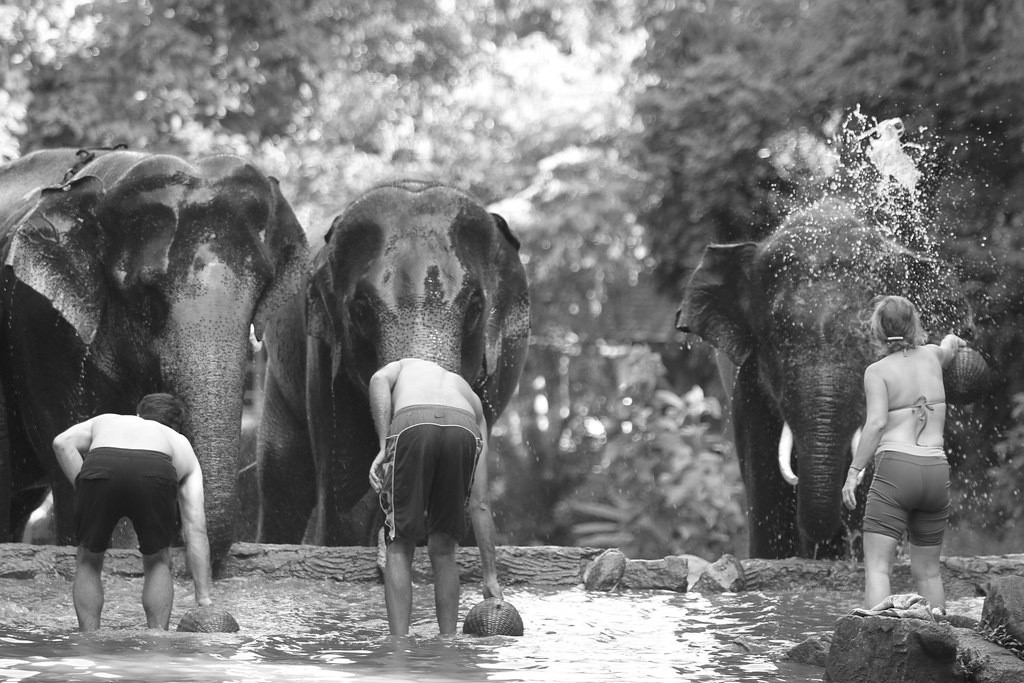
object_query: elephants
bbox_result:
[0,148,531,580]
[673,196,987,562]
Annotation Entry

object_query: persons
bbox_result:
[52,393,213,631]
[21,487,57,545]
[368,357,504,637]
[843,295,967,611]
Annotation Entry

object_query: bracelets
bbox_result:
[850,466,862,472]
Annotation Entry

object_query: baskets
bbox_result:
[463,597,524,636]
[943,346,989,404]
[177,606,240,633]
